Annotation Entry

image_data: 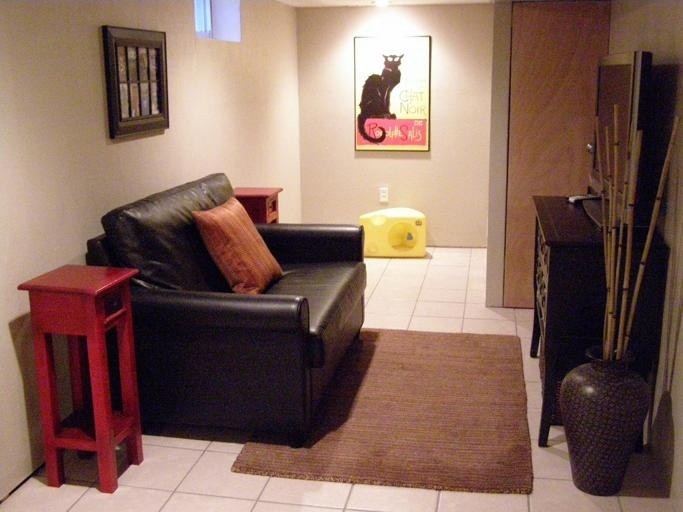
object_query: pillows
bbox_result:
[191,195,284,296]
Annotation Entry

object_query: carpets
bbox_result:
[230,327,534,495]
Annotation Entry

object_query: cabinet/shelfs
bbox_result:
[529,193,672,455]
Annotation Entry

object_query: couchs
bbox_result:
[84,170,367,448]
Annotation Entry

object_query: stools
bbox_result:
[358,206,428,259]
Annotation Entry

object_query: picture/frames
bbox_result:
[101,24,170,140]
[353,34,432,152]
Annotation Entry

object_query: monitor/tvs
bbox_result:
[582,50,650,229]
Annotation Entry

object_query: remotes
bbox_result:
[569,194,602,204]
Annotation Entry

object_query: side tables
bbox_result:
[16,264,144,494]
[233,186,284,225]
